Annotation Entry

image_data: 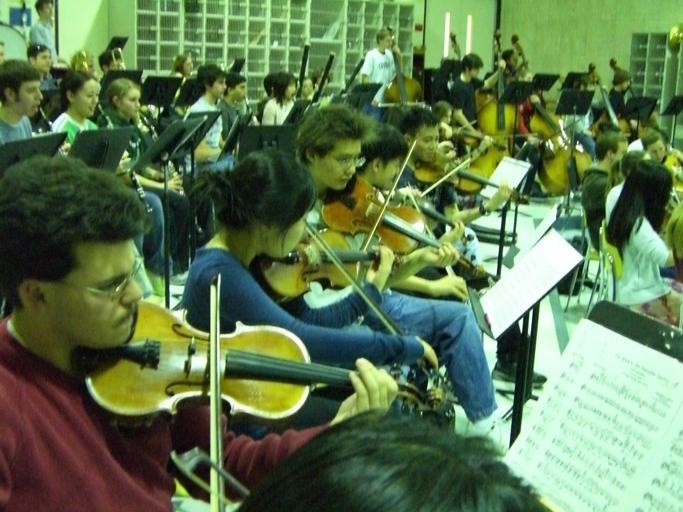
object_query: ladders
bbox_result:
[80,294,442,429]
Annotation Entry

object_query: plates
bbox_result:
[306,0,345,90]
[205,0,307,104]
[628,30,678,117]
[346,0,415,85]
[111,0,204,77]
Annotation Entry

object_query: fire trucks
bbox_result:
[244,97,257,125]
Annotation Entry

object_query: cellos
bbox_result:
[385,26,420,106]
[474,31,518,161]
[524,59,659,194]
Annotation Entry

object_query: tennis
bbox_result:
[524,59,659,194]
[474,31,518,161]
[385,26,420,106]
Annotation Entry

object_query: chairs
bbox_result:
[492,361,547,388]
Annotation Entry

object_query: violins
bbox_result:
[321,173,485,281]
[80,294,442,429]
[413,152,530,205]
[247,230,405,302]
[440,124,506,155]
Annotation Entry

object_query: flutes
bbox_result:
[244,97,257,125]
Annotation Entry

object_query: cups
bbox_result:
[327,154,365,168]
[64,256,144,302]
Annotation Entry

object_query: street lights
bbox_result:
[556,236,589,295]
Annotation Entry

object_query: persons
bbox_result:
[218,74,253,141]
[603,161,682,329]
[98,51,123,82]
[181,65,237,172]
[501,49,529,92]
[26,44,56,90]
[639,127,682,193]
[313,70,329,92]
[361,28,403,85]
[0,153,398,511]
[181,147,438,376]
[0,59,43,145]
[169,55,192,78]
[432,101,454,131]
[261,72,297,127]
[582,132,628,255]
[232,409,554,511]
[608,70,631,119]
[300,78,313,105]
[447,53,507,131]
[357,123,548,399]
[400,108,510,269]
[566,72,608,193]
[106,78,189,281]
[293,105,510,452]
[0,42,4,65]
[255,74,276,124]
[50,71,100,155]
[29,0,57,63]
